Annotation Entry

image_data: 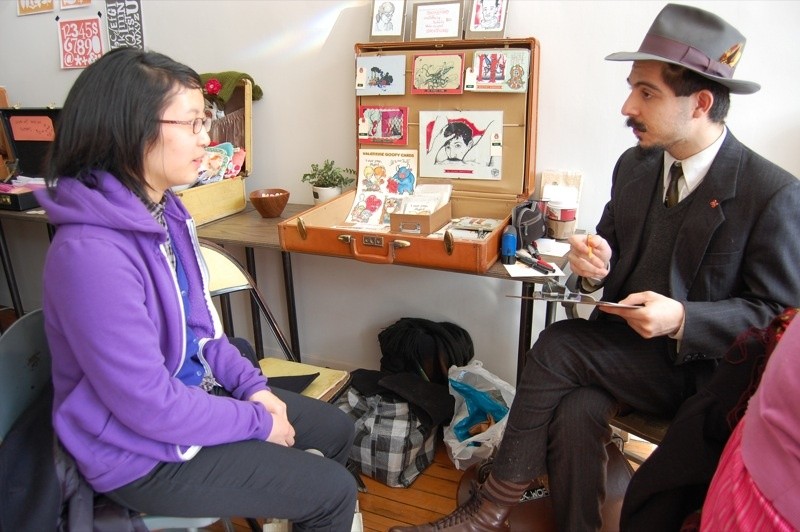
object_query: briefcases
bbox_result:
[279,37,538,276]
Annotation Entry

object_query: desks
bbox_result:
[196,206,591,395]
[0,204,255,337]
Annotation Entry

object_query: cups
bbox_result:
[544,199,577,238]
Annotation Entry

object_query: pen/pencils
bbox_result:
[587,233,592,259]
[515,241,555,275]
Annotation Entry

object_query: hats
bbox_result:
[604,3,761,95]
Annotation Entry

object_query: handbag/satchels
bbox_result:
[443,361,517,471]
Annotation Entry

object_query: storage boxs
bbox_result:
[388,201,451,236]
[0,103,67,211]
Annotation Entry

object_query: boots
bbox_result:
[386,484,512,532]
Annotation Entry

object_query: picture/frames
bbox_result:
[369,0,408,42]
[464,0,509,40]
[410,0,464,42]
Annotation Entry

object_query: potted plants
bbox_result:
[296,161,356,205]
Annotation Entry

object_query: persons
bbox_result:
[29,45,358,532]
[389,3,800,532]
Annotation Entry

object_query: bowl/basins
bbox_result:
[248,188,290,219]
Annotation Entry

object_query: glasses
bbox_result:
[155,118,212,134]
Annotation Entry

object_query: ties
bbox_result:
[662,165,684,208]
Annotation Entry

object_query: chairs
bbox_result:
[0,241,349,532]
[560,267,665,469]
